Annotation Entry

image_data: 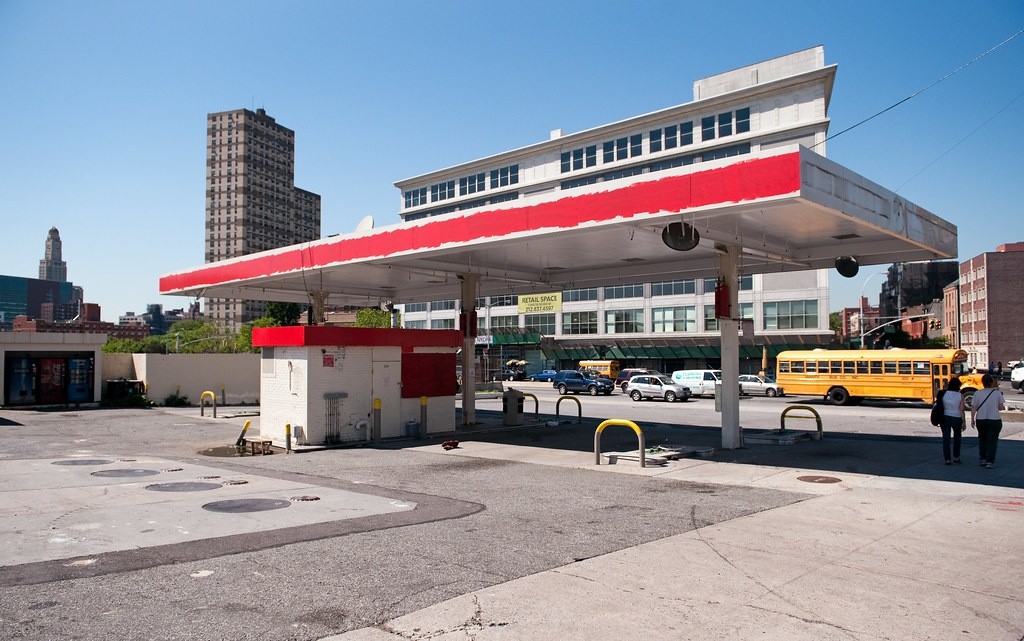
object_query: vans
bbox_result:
[671,370,743,398]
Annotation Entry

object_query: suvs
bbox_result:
[553,371,614,396]
[617,367,663,394]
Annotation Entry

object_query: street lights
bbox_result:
[860,272,889,348]
[150,327,169,355]
[475,298,511,383]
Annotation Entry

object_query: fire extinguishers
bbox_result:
[459,307,466,338]
[469,305,478,338]
[712,281,730,318]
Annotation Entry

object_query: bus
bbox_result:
[776,348,986,411]
[578,359,619,381]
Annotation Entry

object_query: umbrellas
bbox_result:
[507,359,530,371]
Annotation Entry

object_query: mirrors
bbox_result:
[378,298,393,313]
[662,222,700,251]
[835,255,859,278]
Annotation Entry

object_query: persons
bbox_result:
[933,377,966,465]
[971,367,977,374]
[1018,361,1023,367]
[971,375,1006,468]
[550,366,556,370]
[998,362,1004,380]
[989,361,995,378]
[653,379,659,384]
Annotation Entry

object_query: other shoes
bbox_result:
[981,459,986,465]
[953,457,960,463]
[945,460,952,465]
[987,463,992,468]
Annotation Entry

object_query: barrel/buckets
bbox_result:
[406,422,420,437]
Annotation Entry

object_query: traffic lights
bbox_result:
[929,318,935,330]
[935,318,941,330]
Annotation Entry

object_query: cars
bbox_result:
[625,374,692,403]
[1007,358,1024,393]
[529,370,558,383]
[738,375,779,398]
[490,370,526,381]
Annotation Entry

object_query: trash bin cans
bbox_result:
[107,380,144,397]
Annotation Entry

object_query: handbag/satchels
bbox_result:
[931,389,946,426]
